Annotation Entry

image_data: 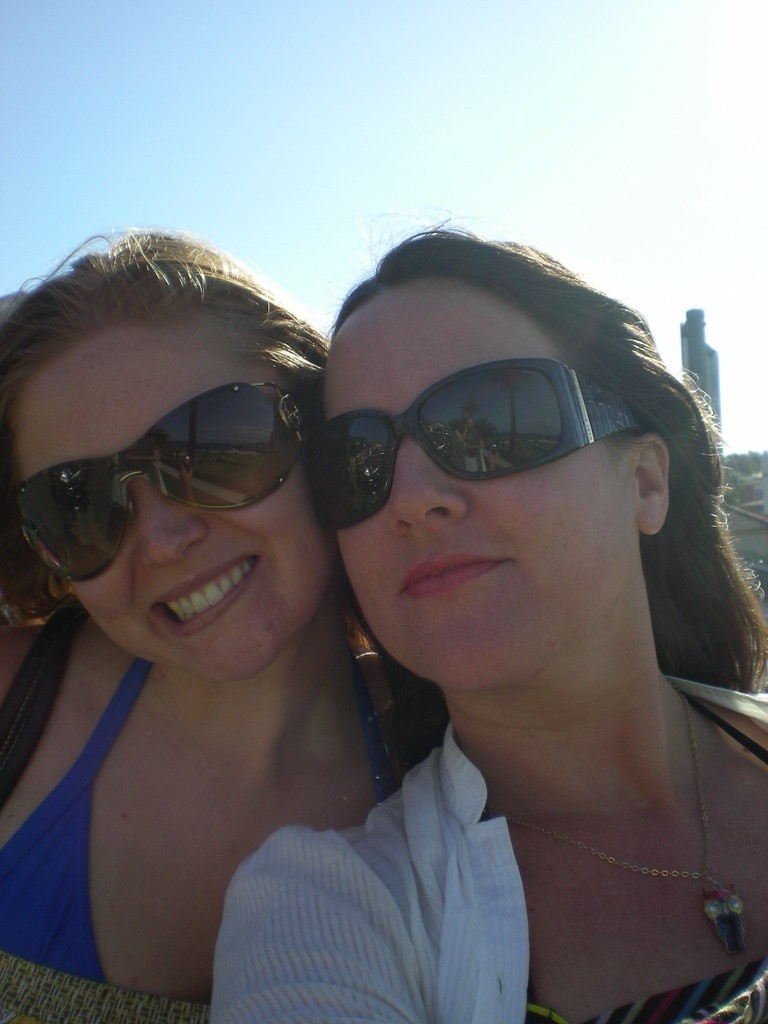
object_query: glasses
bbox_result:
[312,356,639,532]
[11,379,321,583]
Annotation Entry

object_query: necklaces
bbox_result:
[484,679,747,955]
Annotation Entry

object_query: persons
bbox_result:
[488,448,499,471]
[0,233,395,1024]
[462,417,484,472]
[208,227,768,1024]
[179,464,195,503]
